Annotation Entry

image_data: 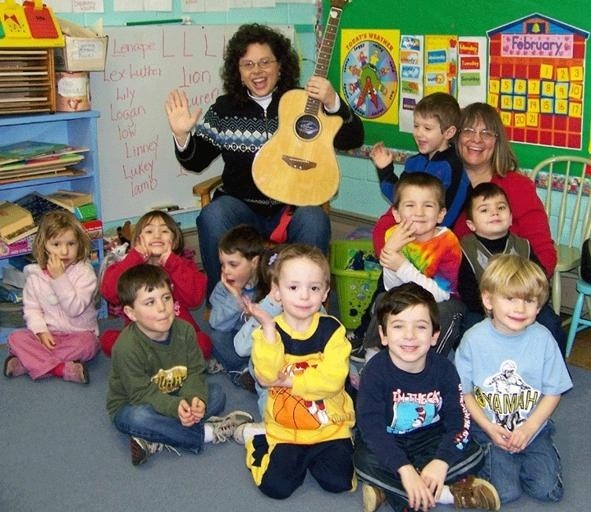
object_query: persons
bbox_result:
[350,177,575,512]
[368,92,471,229]
[373,102,557,280]
[164,23,364,309]
[4,211,102,385]
[100,212,356,501]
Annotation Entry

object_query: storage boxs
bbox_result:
[0,46,56,114]
[55,35,109,74]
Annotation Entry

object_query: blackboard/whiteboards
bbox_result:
[89,23,295,223]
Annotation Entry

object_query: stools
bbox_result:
[566,279,591,358]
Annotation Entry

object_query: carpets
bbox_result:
[0,300,591,512]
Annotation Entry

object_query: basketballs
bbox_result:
[273,388,322,431]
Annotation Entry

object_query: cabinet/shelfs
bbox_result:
[0,110,108,346]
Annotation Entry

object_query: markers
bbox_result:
[151,206,179,213]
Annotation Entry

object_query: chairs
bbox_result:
[195,174,331,321]
[529,156,591,327]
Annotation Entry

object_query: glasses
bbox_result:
[239,57,280,69]
[461,125,498,142]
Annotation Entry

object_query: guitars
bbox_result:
[252,1,346,207]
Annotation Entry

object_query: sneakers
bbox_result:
[205,409,266,446]
[345,332,367,362]
[360,481,386,512]
[129,434,167,467]
[4,356,27,379]
[238,369,257,396]
[448,475,501,512]
[61,361,89,385]
